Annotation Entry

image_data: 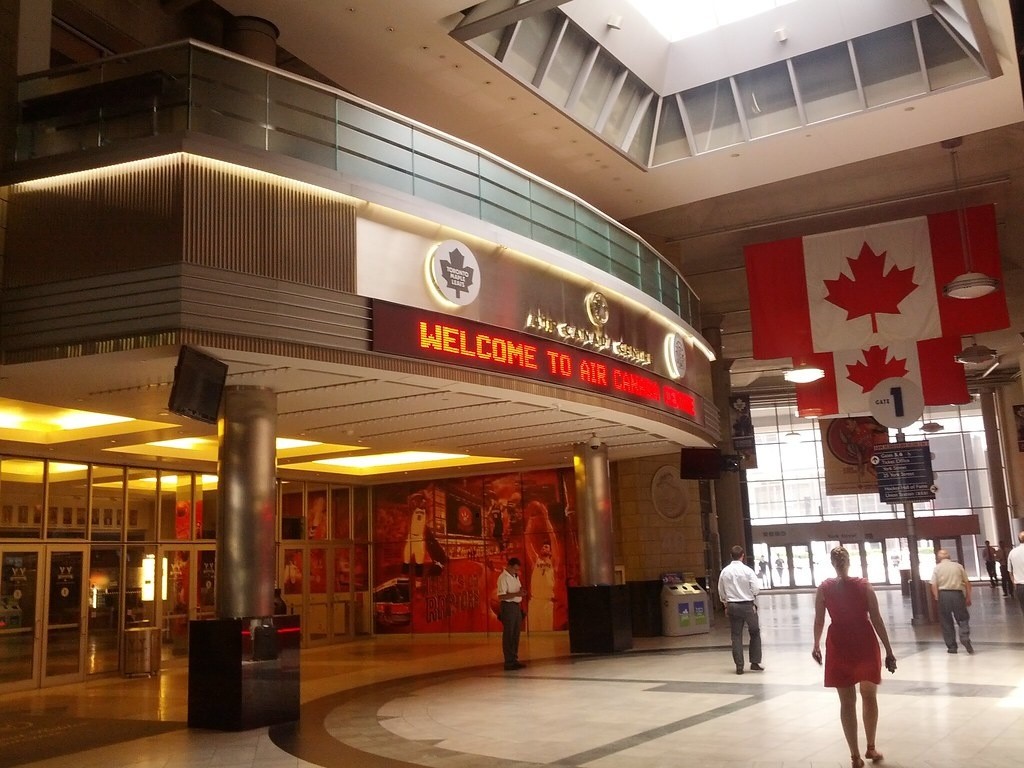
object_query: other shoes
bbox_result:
[948,648,957,653]
[961,640,974,654]
[736,668,744,673]
[750,664,764,670]
[865,744,883,760]
[504,663,526,670]
[851,755,865,768]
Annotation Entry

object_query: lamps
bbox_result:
[941,136,1001,300]
[784,356,825,384]
[955,335,997,364]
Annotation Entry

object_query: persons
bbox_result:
[525,504,558,632]
[930,550,974,653]
[717,545,763,673]
[775,553,784,584]
[1007,531,1024,615]
[982,541,997,587]
[996,541,1013,598]
[812,546,897,768]
[486,499,522,549]
[497,558,527,670]
[759,555,769,589]
[401,490,431,590]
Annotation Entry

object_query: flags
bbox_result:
[742,202,1011,363]
[791,338,971,419]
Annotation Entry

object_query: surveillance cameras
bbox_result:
[589,437,600,450]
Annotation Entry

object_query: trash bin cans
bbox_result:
[901,569,912,595]
[909,579,941,624]
[125,627,162,678]
[661,581,710,637]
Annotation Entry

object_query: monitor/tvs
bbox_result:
[168,344,229,425]
[680,448,720,480]
